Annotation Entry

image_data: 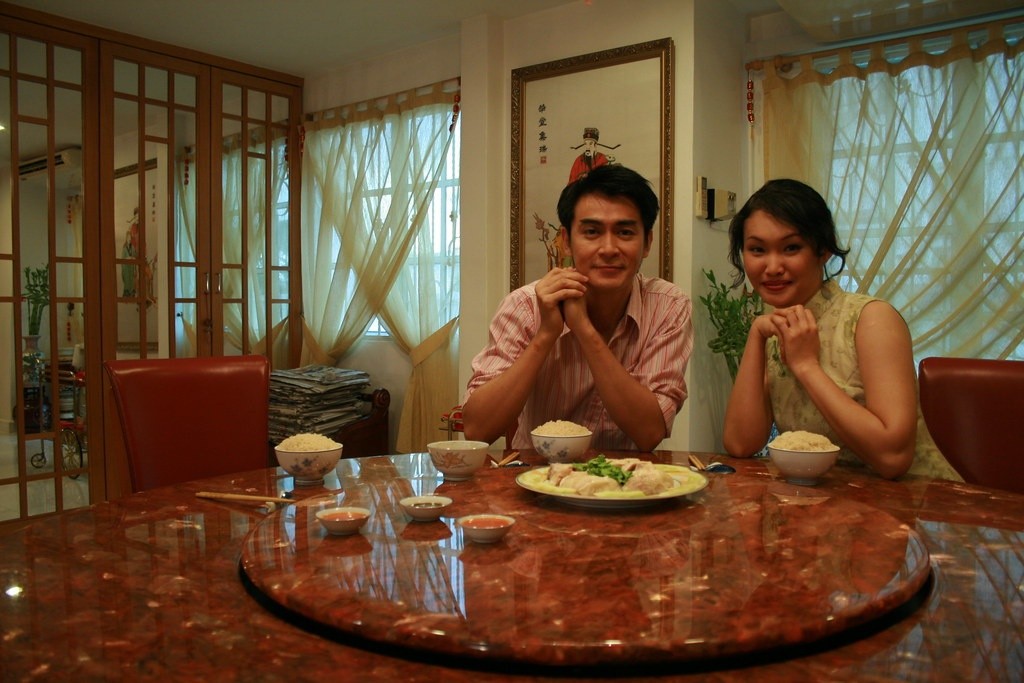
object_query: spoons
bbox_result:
[703,465,736,474]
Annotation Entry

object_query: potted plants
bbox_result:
[12,263,52,435]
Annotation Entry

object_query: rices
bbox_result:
[769,430,838,452]
[276,433,340,451]
[531,419,590,436]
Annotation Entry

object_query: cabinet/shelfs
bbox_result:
[40,349,88,450]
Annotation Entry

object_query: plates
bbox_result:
[515,463,709,508]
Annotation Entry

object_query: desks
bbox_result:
[269,390,390,467]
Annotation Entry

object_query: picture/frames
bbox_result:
[509,37,676,296]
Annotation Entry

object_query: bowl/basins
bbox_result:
[530,431,593,464]
[315,507,371,536]
[426,440,490,481]
[399,496,453,522]
[274,443,344,486]
[457,514,516,543]
[767,443,841,485]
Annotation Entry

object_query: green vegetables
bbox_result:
[570,454,634,484]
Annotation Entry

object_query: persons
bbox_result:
[723,177,966,484]
[460,163,696,453]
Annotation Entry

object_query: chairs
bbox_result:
[919,357,1024,494]
[100,355,273,495]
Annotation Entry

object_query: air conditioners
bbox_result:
[19,149,81,182]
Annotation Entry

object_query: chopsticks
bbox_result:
[689,454,706,470]
[498,451,519,466]
[195,492,297,504]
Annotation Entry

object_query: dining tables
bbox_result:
[0,452,1024,683]
